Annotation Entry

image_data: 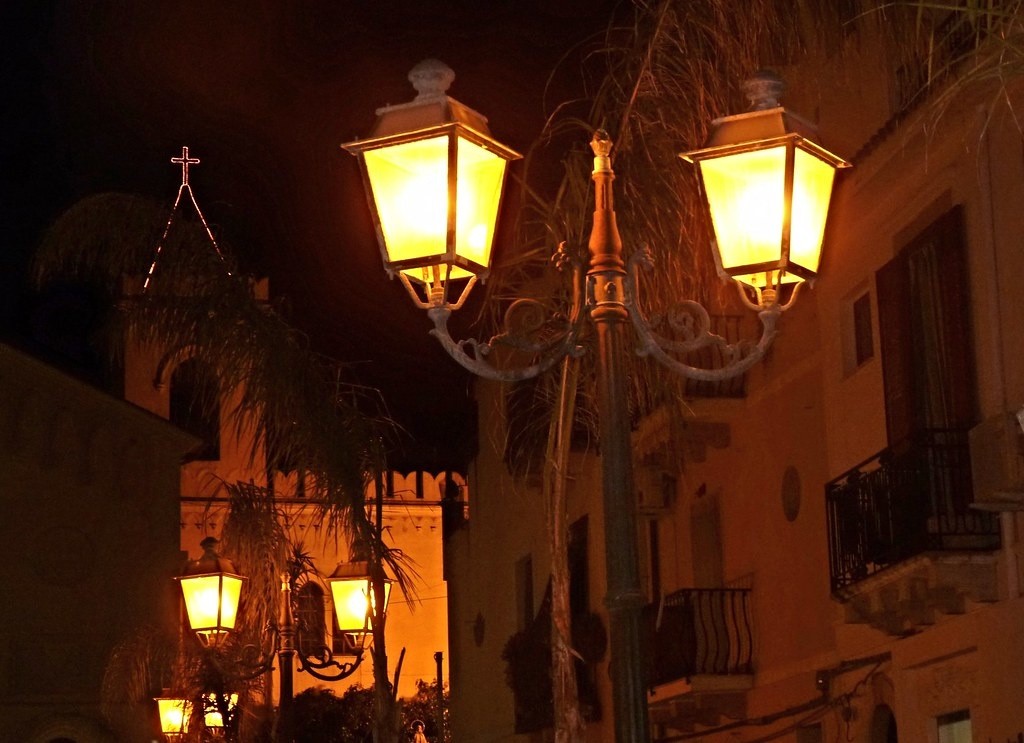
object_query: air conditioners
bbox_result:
[969,411,1024,513]
[634,465,664,508]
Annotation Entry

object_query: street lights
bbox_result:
[170,534,397,743]
[333,49,854,743]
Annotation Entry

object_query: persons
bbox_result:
[412,720,427,743]
[401,724,414,743]
